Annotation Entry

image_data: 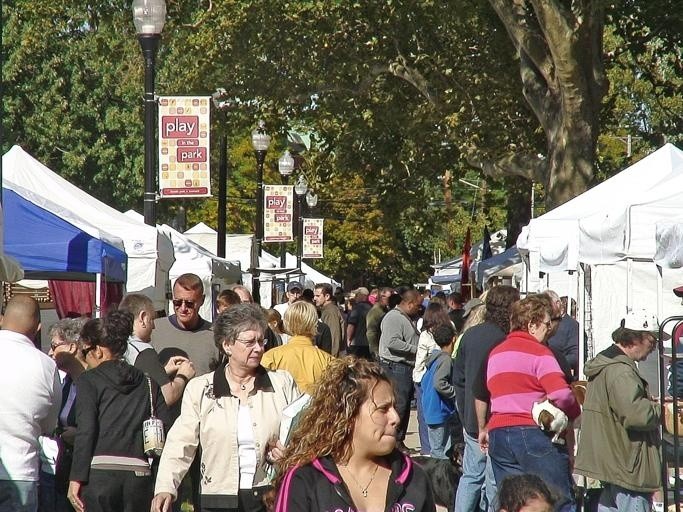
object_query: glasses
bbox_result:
[51,343,68,351]
[235,338,267,347]
[173,300,195,308]
[81,345,95,359]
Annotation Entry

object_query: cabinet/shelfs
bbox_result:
[657,315,683,511]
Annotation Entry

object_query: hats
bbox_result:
[462,299,485,319]
[287,281,303,291]
[621,311,671,341]
[353,287,369,295]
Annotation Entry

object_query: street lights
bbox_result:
[210,81,232,259]
[278,150,293,270]
[131,2,166,227]
[293,174,308,271]
[306,186,318,268]
[248,118,270,306]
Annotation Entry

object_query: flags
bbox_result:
[481,222,492,261]
[459,227,470,301]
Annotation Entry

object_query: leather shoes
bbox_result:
[398,441,415,454]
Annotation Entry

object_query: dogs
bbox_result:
[532,381,588,445]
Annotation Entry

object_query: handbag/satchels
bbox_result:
[143,420,165,458]
[36,427,63,479]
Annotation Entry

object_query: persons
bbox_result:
[452,286,670,512]
[0,273,230,512]
[215,281,467,512]
